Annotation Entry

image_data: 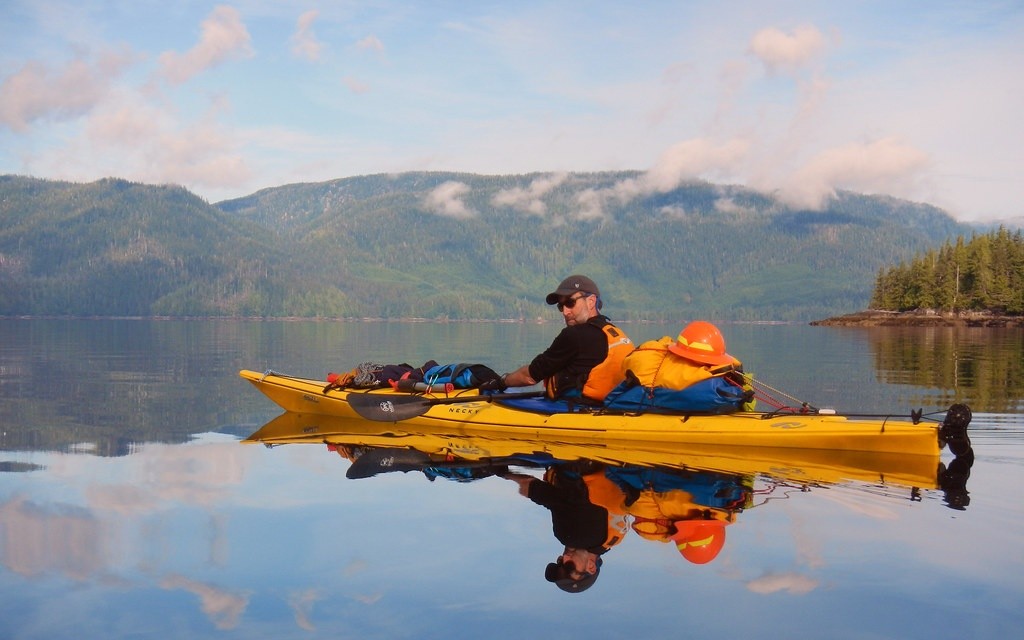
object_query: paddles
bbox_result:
[344,449,515,480]
[348,389,545,424]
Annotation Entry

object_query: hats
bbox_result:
[546,275,599,305]
[544,562,599,593]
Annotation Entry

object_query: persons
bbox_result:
[489,465,631,593]
[479,275,636,405]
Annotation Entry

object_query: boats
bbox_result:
[239,411,975,562]
[238,366,973,453]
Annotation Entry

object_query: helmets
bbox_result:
[674,521,729,564]
[668,321,734,365]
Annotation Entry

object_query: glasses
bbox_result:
[556,556,576,572]
[557,295,598,312]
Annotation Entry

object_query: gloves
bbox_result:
[479,378,508,403]
